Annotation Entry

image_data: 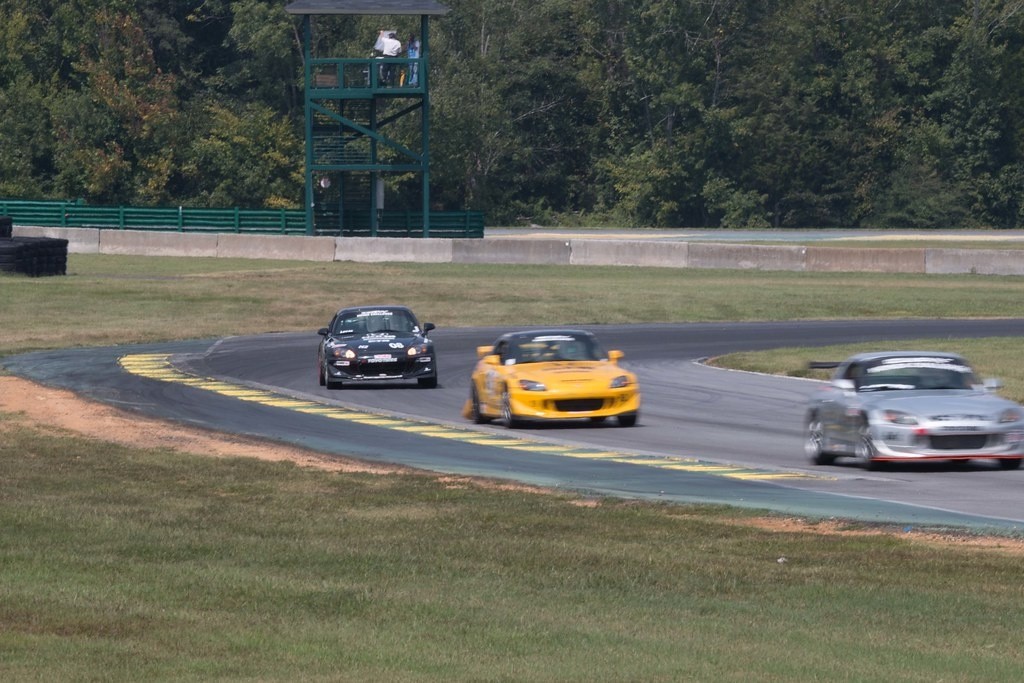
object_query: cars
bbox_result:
[801,350,1023,469]
[314,304,442,389]
[464,330,640,429]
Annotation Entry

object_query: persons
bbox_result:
[406,32,421,87]
[379,30,401,85]
[399,69,408,87]
